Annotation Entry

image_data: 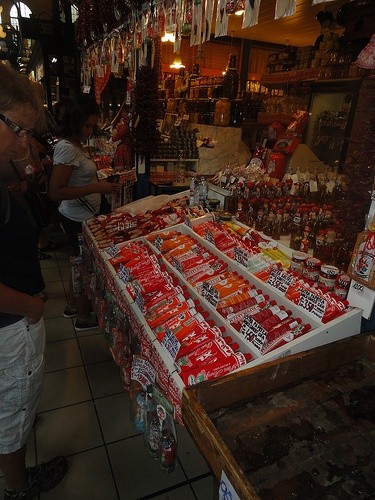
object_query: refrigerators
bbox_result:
[301,76,375,175]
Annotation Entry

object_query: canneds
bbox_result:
[291,252,351,299]
[219,214,232,222]
[160,76,245,127]
[209,199,220,210]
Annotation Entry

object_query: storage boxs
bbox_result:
[181,329,375,500]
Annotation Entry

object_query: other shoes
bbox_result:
[75,314,102,331]
[63,305,80,318]
[39,235,59,251]
[37,251,50,260]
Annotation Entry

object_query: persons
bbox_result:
[48,93,123,331]
[0,81,72,302]
[0,61,68,500]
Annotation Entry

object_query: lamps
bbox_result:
[170,54,187,70]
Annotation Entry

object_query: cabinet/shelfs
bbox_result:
[96,213,365,388]
[151,71,364,190]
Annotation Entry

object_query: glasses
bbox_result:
[0,112,31,137]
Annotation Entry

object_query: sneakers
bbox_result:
[4,456,69,500]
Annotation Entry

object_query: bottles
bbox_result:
[104,159,374,385]
[158,44,312,128]
